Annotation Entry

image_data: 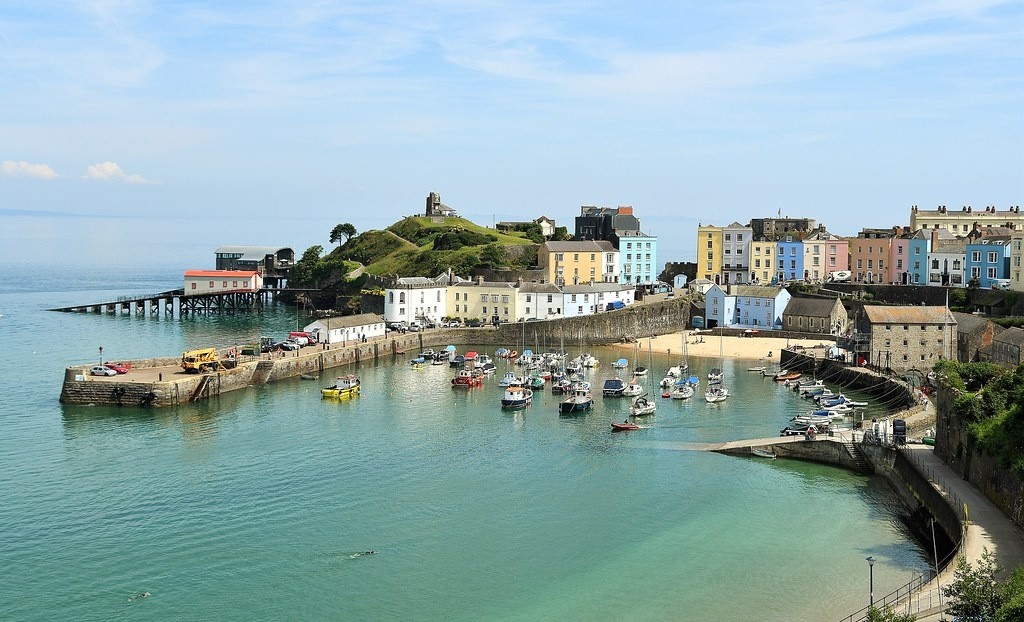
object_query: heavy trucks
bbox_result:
[770,276,789,286]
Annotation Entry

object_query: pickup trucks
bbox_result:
[746,280,763,287]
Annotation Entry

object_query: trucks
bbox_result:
[608,301,625,310]
[991,278,1010,292]
[824,270,852,283]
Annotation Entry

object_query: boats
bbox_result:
[411,345,497,385]
[748,366,868,435]
[321,374,361,396]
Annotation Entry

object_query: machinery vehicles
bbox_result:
[261,337,274,353]
[180,348,237,373]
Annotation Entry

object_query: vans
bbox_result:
[668,292,674,299]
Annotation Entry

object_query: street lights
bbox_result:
[912,365,915,391]
[866,556,877,607]
[99,346,103,366]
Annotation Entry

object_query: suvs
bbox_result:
[440,321,460,328]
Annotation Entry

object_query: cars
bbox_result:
[274,332,316,351]
[526,318,544,321]
[493,320,509,327]
[465,321,485,327]
[90,364,129,376]
[386,323,421,333]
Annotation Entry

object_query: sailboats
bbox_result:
[495,317,600,411]
[603,320,729,430]
